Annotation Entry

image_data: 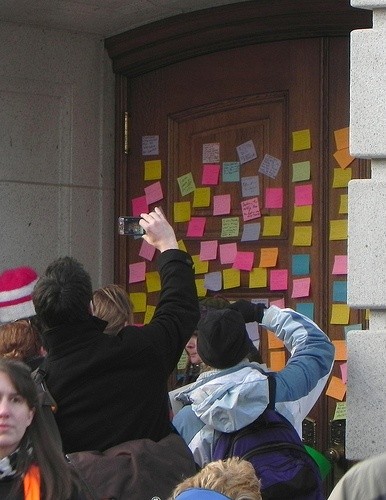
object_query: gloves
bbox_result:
[230,300,267,324]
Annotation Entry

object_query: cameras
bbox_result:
[118,216,146,237]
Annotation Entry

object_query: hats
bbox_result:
[197,308,251,370]
[1,265,38,324]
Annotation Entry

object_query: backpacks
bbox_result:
[209,373,325,500]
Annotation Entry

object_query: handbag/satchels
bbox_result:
[61,429,202,500]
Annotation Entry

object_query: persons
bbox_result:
[31,207,201,473]
[0,269,386,500]
